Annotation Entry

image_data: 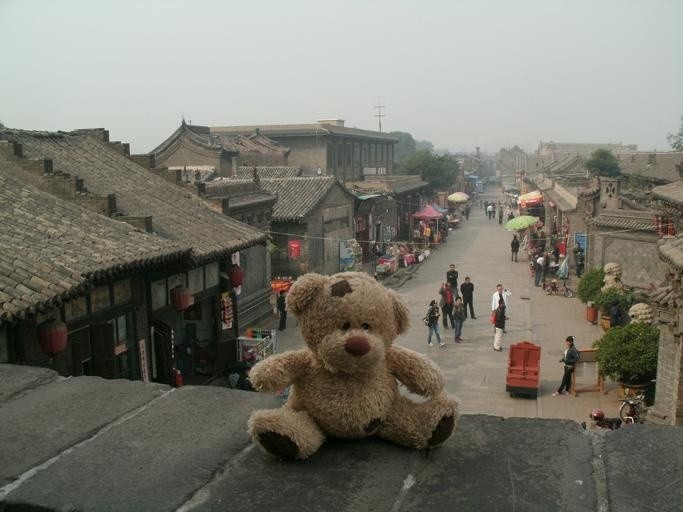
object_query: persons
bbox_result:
[373,197,470,256]
[422,264,511,352]
[481,198,586,287]
[553,335,579,398]
[277,290,288,331]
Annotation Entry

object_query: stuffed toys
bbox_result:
[246,269,461,462]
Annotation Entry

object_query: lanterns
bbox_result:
[229,265,244,288]
[37,317,67,357]
[169,285,193,313]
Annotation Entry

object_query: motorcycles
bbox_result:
[588,391,640,430]
[542,276,573,298]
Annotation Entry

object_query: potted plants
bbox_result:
[591,285,626,332]
[590,320,660,402]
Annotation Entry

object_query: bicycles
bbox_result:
[617,388,648,420]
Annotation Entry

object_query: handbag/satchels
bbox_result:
[423,316,434,327]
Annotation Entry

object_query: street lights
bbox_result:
[373,103,385,132]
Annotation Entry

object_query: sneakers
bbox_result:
[427,315,507,351]
